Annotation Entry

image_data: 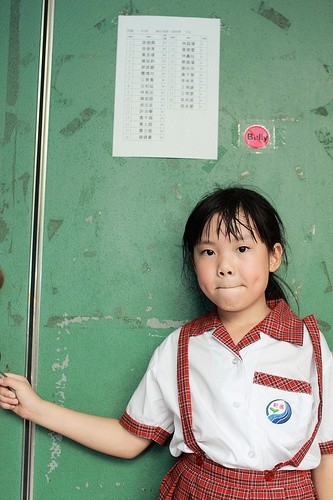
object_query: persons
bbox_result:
[1,186,333,500]
